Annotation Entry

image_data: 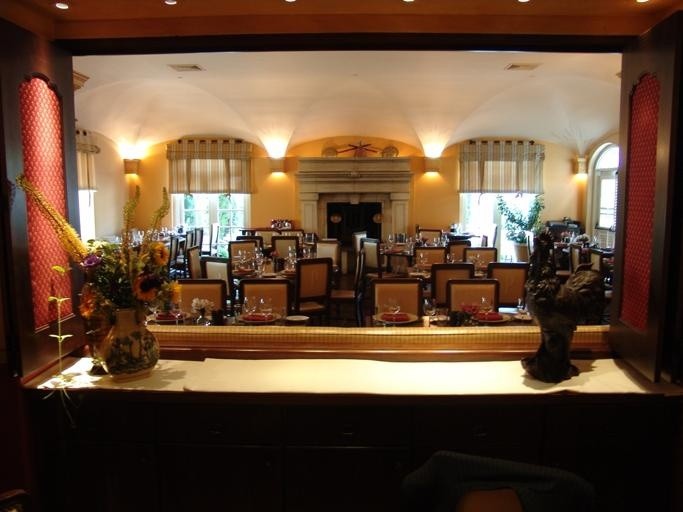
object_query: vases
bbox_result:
[100,310,162,382]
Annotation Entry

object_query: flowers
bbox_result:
[11,171,184,319]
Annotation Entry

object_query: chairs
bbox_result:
[416,224,443,243]
[462,247,498,277]
[236,229,318,251]
[366,277,423,321]
[316,239,342,289]
[487,263,530,308]
[446,278,500,314]
[228,241,257,296]
[272,236,299,260]
[431,262,476,306]
[574,247,604,275]
[238,278,291,319]
[481,235,489,246]
[291,257,334,324]
[555,245,583,280]
[202,222,220,255]
[200,256,238,317]
[342,249,350,275]
[166,228,204,279]
[354,233,379,326]
[174,279,227,316]
[380,239,471,275]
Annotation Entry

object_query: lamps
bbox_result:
[266,154,287,176]
[571,156,588,176]
[422,155,443,176]
[122,156,141,175]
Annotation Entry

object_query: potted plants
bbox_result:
[494,189,547,261]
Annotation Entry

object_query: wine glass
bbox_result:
[517,297,529,323]
[418,252,430,276]
[148,298,183,326]
[286,244,297,272]
[243,295,275,321]
[119,227,177,246]
[236,245,264,272]
[302,232,315,256]
[480,295,492,320]
[385,297,400,327]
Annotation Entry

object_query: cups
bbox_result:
[446,252,455,264]
[504,254,513,263]
[380,232,450,256]
[436,308,450,322]
[421,298,437,316]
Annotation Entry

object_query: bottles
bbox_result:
[271,215,296,231]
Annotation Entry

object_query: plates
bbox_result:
[421,314,450,322]
[470,312,509,323]
[261,272,277,278]
[144,311,190,323]
[285,314,309,322]
[235,314,282,324]
[371,311,417,325]
[513,315,533,323]
